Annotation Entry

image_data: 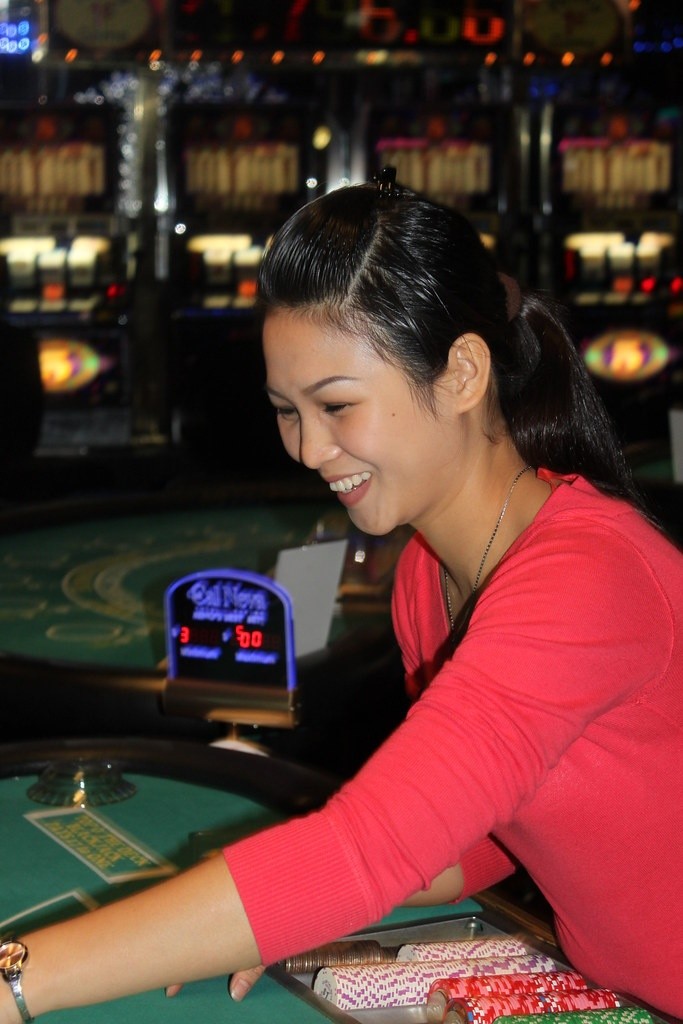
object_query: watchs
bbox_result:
[0,933,31,1024]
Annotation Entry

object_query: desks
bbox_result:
[0,737,683,1023]
[0,481,406,781]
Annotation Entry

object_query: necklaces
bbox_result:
[444,464,532,642]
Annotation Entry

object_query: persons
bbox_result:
[0,178,682,1024]
